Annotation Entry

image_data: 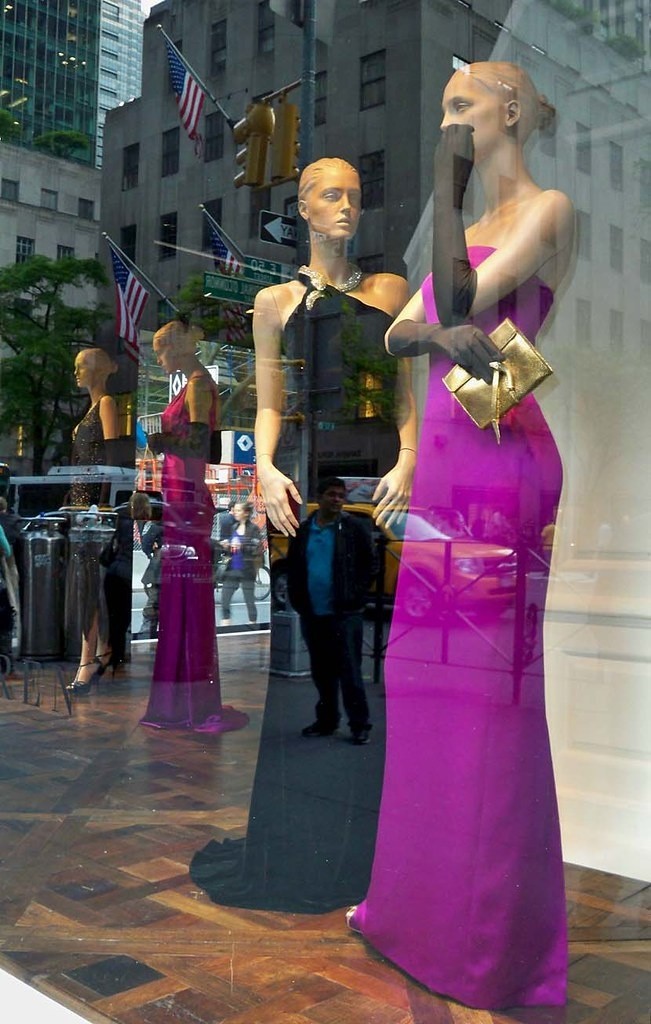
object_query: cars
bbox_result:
[112,500,185,528]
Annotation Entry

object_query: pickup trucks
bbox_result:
[267,498,528,614]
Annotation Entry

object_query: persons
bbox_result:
[140,521,162,635]
[189,158,418,916]
[220,501,239,542]
[140,322,251,735]
[221,503,263,627]
[346,64,576,1007]
[285,477,378,745]
[65,348,122,697]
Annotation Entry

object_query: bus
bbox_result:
[0,462,199,518]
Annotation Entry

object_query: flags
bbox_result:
[108,244,148,367]
[204,218,241,276]
[164,41,206,143]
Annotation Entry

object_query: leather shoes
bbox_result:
[351,728,369,743]
[301,720,338,737]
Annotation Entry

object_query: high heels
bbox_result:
[65,648,117,694]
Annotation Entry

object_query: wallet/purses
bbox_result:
[442,318,553,445]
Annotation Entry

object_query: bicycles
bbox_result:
[211,548,273,609]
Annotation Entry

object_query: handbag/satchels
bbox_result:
[99,528,117,566]
[215,546,232,588]
